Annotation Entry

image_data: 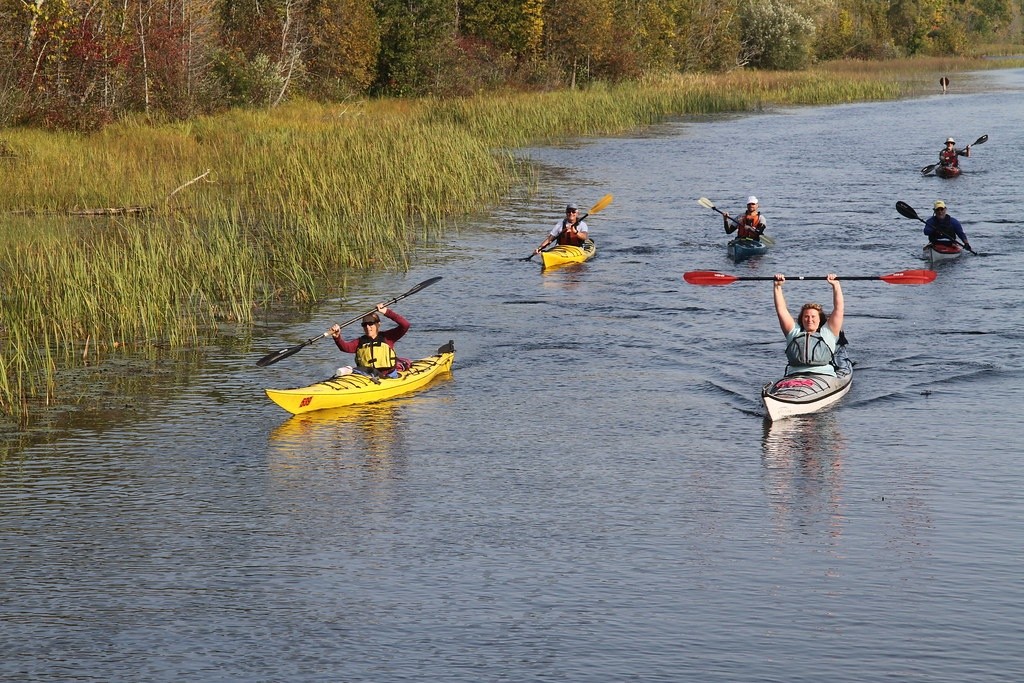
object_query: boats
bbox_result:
[540,238,595,269]
[923,240,963,264]
[763,415,805,466]
[760,332,854,421]
[271,397,416,440]
[726,238,767,263]
[935,163,962,178]
[264,341,455,416]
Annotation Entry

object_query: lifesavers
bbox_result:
[940,78,949,86]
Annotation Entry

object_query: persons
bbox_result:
[723,196,766,244]
[773,272,844,380]
[939,137,971,169]
[923,200,972,252]
[533,203,589,256]
[329,303,410,379]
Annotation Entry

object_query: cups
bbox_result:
[336,365,353,376]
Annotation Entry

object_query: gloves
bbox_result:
[963,243,971,251]
[936,229,943,236]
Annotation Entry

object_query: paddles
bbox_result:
[255,275,444,368]
[895,200,978,256]
[698,196,777,247]
[525,193,614,262]
[683,269,937,286]
[921,134,988,174]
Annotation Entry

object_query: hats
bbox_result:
[934,201,945,210]
[944,137,955,145]
[747,196,758,204]
[566,203,578,210]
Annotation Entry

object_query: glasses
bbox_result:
[361,322,378,327]
[947,142,953,144]
[747,203,755,205]
[566,210,577,213]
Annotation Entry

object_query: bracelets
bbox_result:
[574,230,578,235]
